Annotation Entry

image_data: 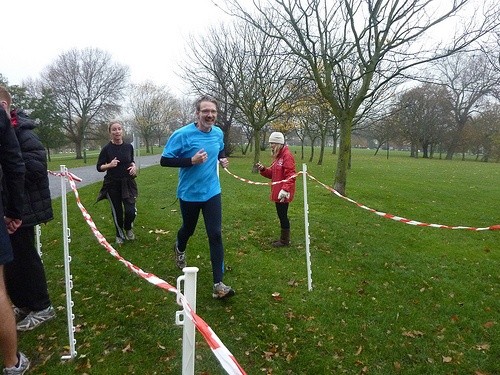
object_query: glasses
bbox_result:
[199,110,217,115]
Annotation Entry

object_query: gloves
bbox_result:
[278,189,290,202]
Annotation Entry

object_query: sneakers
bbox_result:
[212,281,236,298]
[3,352,30,375]
[116,227,135,245]
[12,304,56,331]
[173,241,187,269]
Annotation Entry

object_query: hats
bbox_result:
[269,131,285,144]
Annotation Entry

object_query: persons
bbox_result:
[93,122,139,245]
[1,86,57,331]
[2,104,31,374]
[257,132,297,248]
[160,94,237,299]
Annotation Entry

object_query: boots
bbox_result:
[272,228,290,248]
